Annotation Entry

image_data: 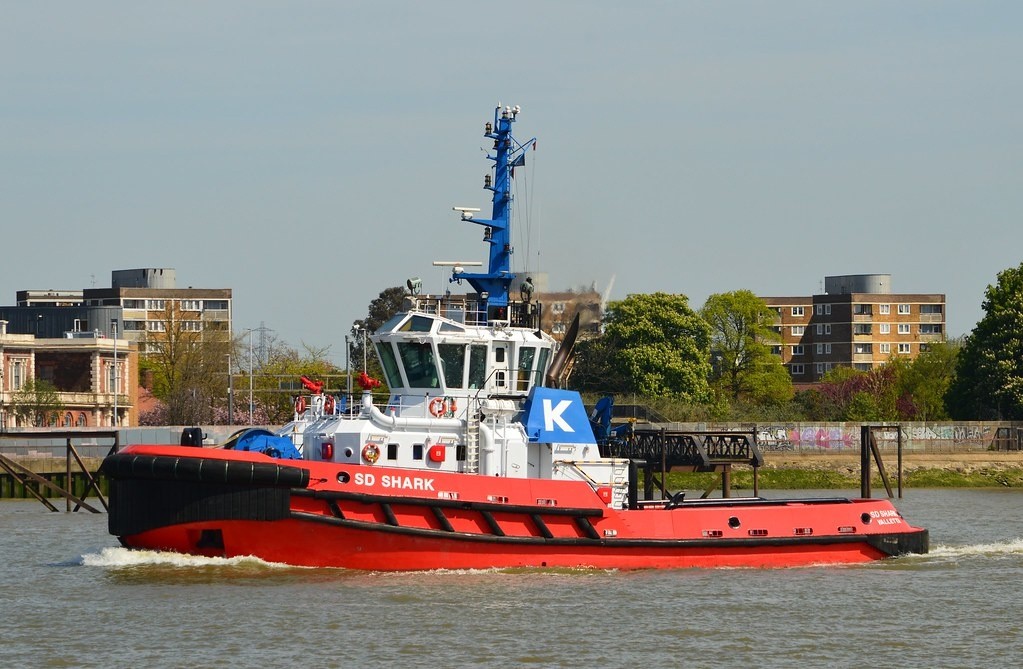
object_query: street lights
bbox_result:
[223,353,233,425]
[109,321,118,426]
[356,328,367,376]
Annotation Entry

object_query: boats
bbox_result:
[101,99,931,572]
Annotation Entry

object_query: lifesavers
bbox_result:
[324,395,333,415]
[362,444,381,462]
[295,396,306,412]
[430,398,447,417]
